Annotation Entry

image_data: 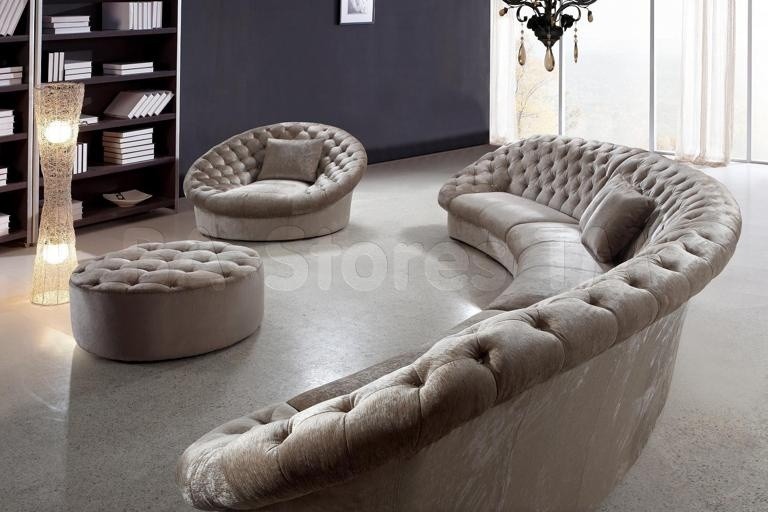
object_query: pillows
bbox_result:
[579,181,658,264]
[257,137,325,183]
[578,173,643,235]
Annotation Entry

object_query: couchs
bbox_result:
[175,133,743,511]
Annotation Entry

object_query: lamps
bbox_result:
[30,81,87,307]
[499,0,597,72]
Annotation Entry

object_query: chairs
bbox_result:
[182,121,368,243]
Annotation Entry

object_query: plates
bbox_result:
[103,189,152,208]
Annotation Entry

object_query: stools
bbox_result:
[68,239,266,364]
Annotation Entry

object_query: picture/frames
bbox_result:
[336,0,376,26]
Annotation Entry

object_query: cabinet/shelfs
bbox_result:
[31,0,182,246]
[0,0,36,248]
